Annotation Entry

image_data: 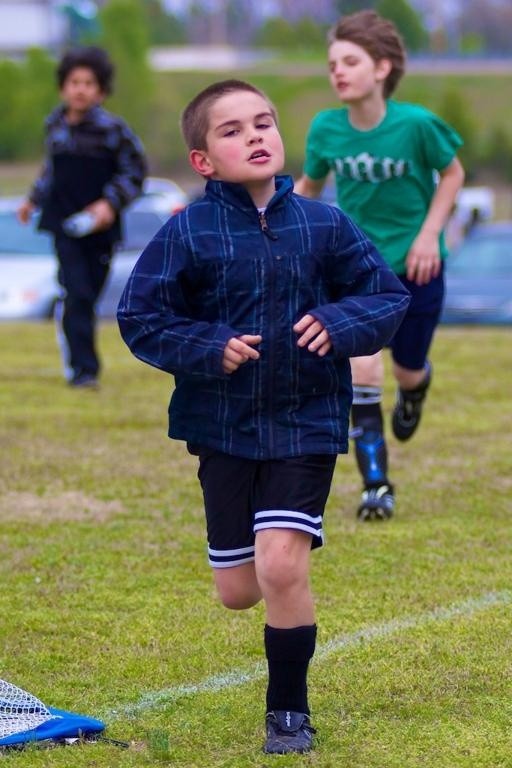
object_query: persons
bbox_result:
[287,8,467,527]
[113,76,419,760]
[17,44,149,391]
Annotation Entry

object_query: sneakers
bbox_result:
[259,705,319,756]
[389,357,433,443]
[355,480,397,523]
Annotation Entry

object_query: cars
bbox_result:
[430,223,512,326]
[0,177,189,327]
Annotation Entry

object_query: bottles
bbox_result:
[62,210,96,238]
